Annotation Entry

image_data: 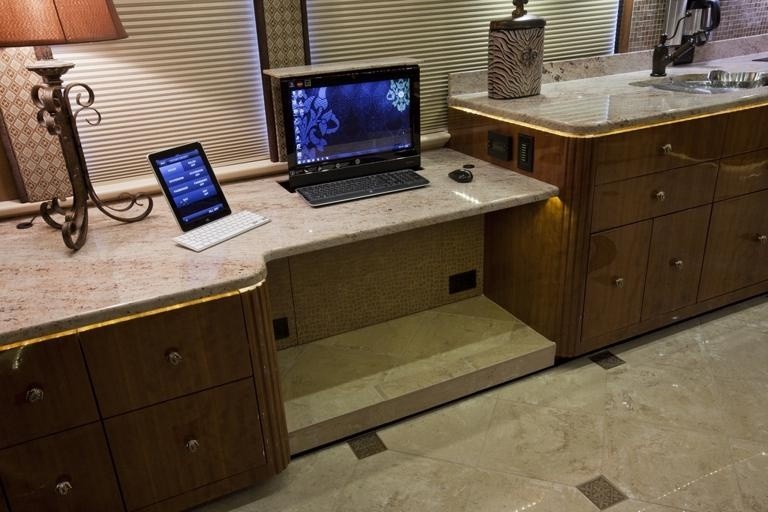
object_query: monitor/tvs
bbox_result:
[274,64,421,174]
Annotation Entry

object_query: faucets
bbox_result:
[648,31,709,78]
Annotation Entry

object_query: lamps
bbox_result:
[0,1,155,256]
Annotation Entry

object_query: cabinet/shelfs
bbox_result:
[0,146,558,512]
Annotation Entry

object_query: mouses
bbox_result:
[447,168,473,183]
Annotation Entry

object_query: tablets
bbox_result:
[147,141,232,231]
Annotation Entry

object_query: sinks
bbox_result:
[630,64,768,96]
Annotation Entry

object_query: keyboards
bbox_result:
[171,210,272,252]
[295,169,431,207]
